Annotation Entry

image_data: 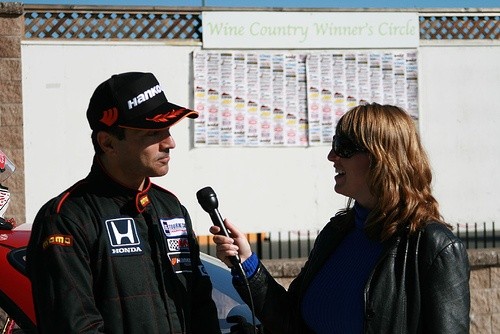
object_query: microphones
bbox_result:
[196,185,241,267]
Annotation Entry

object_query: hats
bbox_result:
[87,72,198,129]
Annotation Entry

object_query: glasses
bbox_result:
[333,135,369,158]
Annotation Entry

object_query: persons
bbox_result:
[210,102,469,334]
[29,72,221,334]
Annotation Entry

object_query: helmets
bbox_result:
[0,150,17,230]
